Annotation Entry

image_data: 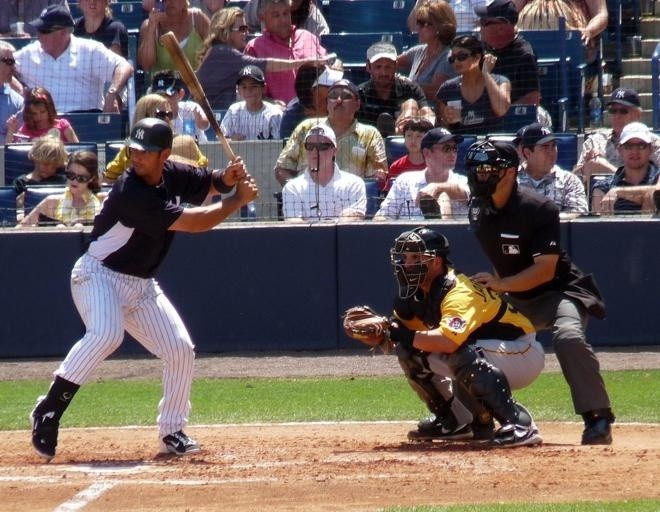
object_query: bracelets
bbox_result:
[388,328,417,347]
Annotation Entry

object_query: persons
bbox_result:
[411,140,616,446]
[341,225,545,448]
[27,115,259,461]
[2,1,660,230]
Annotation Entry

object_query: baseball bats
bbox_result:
[161,30,237,163]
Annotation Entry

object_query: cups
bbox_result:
[447,100,462,122]
[12,134,30,143]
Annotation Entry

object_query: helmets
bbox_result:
[390,225,450,300]
[466,138,521,215]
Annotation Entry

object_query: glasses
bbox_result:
[63,172,91,183]
[156,109,174,121]
[303,142,332,152]
[230,24,248,33]
[326,91,357,100]
[622,140,650,150]
[0,58,15,64]
[35,26,65,34]
[446,53,475,64]
[473,16,507,26]
[607,107,633,115]
[415,18,433,29]
[431,144,459,154]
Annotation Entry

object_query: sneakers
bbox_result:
[163,431,200,455]
[407,422,473,440]
[582,408,614,444]
[485,425,542,448]
[29,403,58,458]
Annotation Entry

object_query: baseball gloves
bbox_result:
[341,306,396,355]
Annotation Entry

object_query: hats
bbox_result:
[328,79,360,99]
[619,122,651,144]
[30,4,74,31]
[522,121,563,145]
[474,1,518,25]
[122,117,173,152]
[420,127,464,149]
[366,41,397,64]
[312,65,344,86]
[151,69,187,96]
[606,87,642,107]
[304,123,337,149]
[236,65,265,85]
[449,34,484,59]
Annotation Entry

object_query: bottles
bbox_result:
[247,201,256,222]
[183,108,195,137]
[589,93,602,129]
